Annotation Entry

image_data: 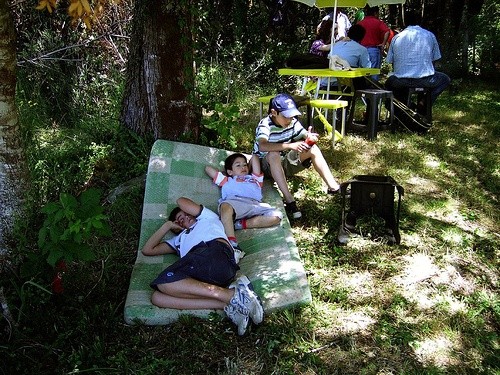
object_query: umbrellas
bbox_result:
[294,0,408,129]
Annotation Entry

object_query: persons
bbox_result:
[310,4,451,118]
[205,152,283,263]
[253,93,351,218]
[142,197,264,337]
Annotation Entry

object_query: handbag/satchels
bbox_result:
[286,53,351,71]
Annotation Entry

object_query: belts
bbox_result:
[368,46,381,49]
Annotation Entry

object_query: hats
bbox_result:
[272,94,302,118]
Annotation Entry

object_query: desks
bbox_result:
[277,67,381,130]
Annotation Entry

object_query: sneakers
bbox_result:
[230,240,246,265]
[285,201,302,219]
[327,188,351,199]
[223,303,249,336]
[234,220,244,231]
[229,274,265,325]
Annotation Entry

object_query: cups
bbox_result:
[304,136,317,153]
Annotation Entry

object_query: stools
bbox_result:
[349,89,393,140]
[259,93,311,124]
[305,100,348,149]
[338,173,405,247]
[395,86,432,130]
[316,87,354,121]
